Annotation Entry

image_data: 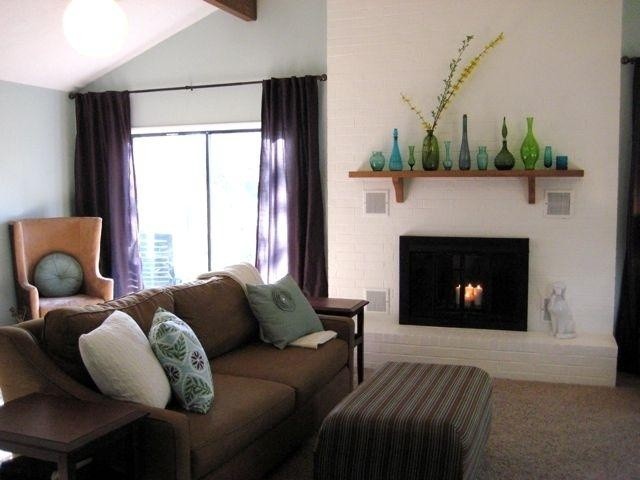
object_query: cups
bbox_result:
[557,156,568,170]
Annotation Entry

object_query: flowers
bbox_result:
[399,29,507,161]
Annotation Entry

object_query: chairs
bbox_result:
[7,216,115,321]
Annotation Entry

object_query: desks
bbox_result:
[301,296,369,386]
[0,392,149,479]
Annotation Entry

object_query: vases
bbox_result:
[368,110,553,171]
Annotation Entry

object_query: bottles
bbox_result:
[370,113,551,171]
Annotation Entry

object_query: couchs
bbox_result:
[0,261,355,479]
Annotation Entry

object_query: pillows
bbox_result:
[244,271,325,351]
[79,308,172,414]
[147,301,215,417]
[33,251,84,299]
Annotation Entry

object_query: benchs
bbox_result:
[308,356,497,480]
